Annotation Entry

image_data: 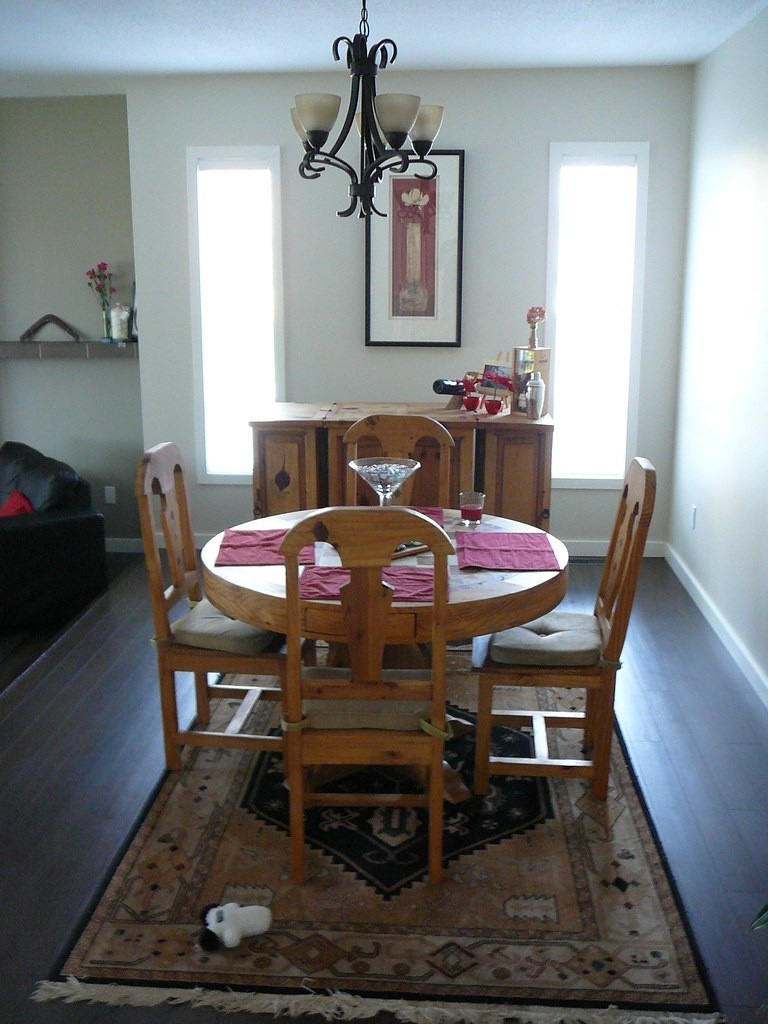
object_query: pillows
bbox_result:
[0,490,33,517]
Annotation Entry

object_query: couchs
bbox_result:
[0,440,107,627]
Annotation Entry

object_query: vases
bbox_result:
[100,310,112,339]
[484,400,501,414]
[462,396,480,411]
[529,324,539,348]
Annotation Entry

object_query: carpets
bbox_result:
[31,650,727,1024]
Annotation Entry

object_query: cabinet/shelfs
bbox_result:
[249,402,554,534]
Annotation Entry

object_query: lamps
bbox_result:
[292,0,445,217]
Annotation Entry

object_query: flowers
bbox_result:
[86,262,117,338]
[528,305,546,324]
[484,370,516,401]
[462,378,481,394]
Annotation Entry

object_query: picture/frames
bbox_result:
[363,148,465,348]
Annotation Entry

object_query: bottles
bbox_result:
[432,378,464,395]
[525,371,545,420]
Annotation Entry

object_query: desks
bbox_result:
[200,506,569,804]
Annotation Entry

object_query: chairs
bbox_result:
[277,505,457,886]
[343,414,456,512]
[135,442,316,781]
[470,456,657,800]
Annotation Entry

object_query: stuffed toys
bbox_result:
[194,902,271,952]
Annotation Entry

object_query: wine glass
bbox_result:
[349,458,422,507]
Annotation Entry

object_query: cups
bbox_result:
[462,396,480,411]
[485,399,502,414]
[459,492,485,525]
